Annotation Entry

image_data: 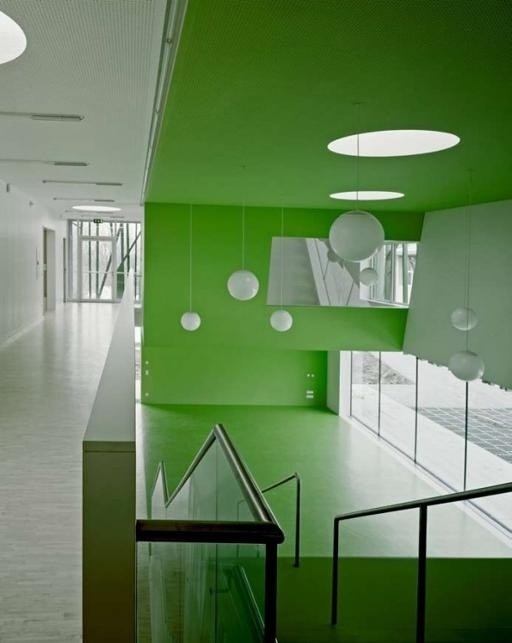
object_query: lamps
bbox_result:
[447,199,486,381]
[360,209,378,286]
[449,198,479,332]
[181,204,202,332]
[226,201,260,302]
[328,104,386,263]
[270,208,293,332]
